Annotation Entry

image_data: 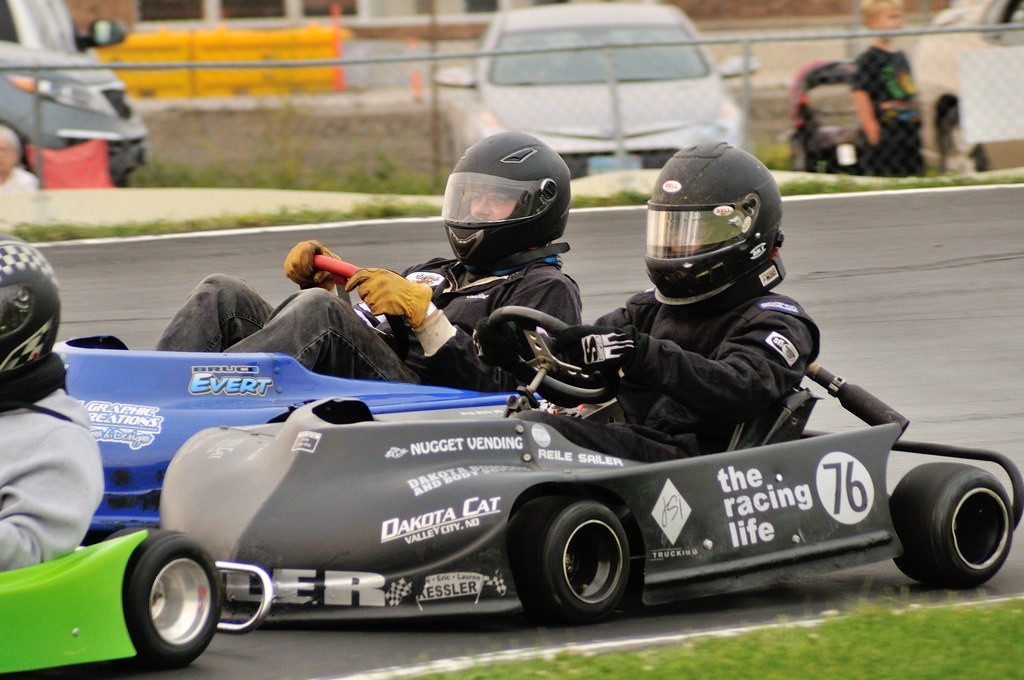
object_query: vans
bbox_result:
[0,0,151,190]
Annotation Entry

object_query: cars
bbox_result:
[908,0,1024,173]
[429,2,763,177]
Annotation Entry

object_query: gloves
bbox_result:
[345,267,432,329]
[283,239,343,292]
[548,324,641,373]
[471,318,520,369]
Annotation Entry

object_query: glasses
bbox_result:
[457,188,517,204]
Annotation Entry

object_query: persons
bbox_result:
[0,233,105,571]
[0,125,39,190]
[472,140,821,462]
[154,129,584,393]
[851,1,927,177]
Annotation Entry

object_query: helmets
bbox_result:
[440,131,573,274]
[1,233,60,372]
[642,140,787,317]
